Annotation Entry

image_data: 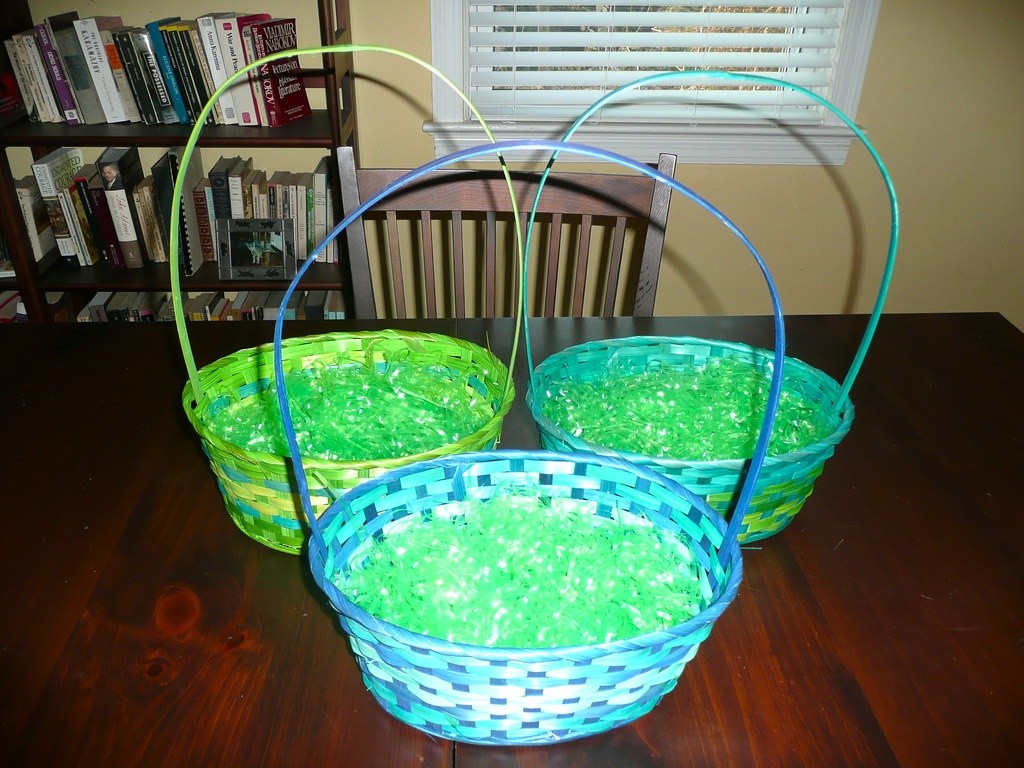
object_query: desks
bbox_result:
[0,312,1024,768]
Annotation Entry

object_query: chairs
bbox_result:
[331,145,678,319]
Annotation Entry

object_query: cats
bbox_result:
[246,240,265,264]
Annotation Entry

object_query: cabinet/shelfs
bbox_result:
[0,0,361,323]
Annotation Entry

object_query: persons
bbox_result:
[100,165,122,191]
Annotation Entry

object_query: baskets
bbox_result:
[522,71,897,546]
[170,44,523,556]
[273,138,785,747]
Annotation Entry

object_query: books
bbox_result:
[0,9,313,128]
[0,290,353,322]
[0,260,16,278]
[15,142,342,268]
